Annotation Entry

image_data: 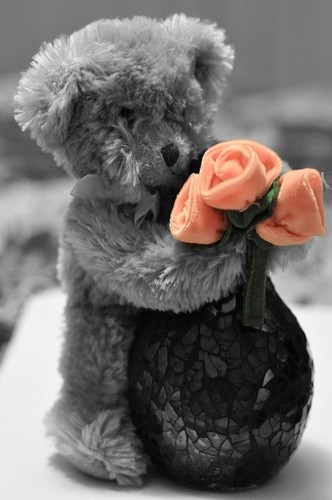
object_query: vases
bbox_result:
[127,271,313,489]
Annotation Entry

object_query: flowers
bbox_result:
[169,132,326,324]
